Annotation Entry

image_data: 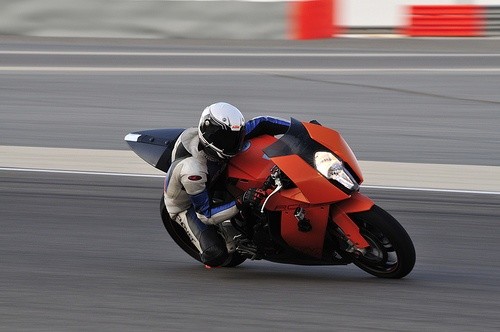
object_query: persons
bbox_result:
[162,102,324,268]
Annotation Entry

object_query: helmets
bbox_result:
[197,102,246,164]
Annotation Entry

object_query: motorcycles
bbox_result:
[124,117,416,279]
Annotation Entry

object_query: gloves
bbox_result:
[236,188,268,211]
[310,120,322,126]
